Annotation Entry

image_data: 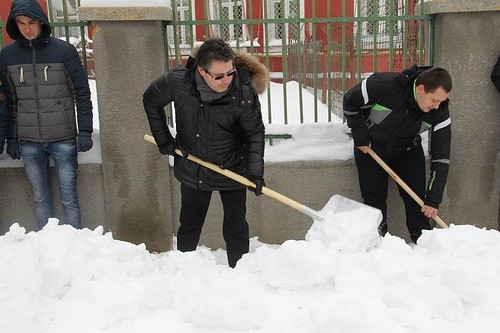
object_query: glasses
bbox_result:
[203,63,237,80]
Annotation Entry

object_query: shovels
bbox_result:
[144,134,383,254]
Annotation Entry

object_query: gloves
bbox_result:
[248,178,266,195]
[6,143,21,160]
[159,137,181,157]
[78,134,93,152]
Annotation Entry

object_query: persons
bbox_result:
[0,0,94,231]
[0,80,9,154]
[141,35,265,268]
[342,63,453,245]
